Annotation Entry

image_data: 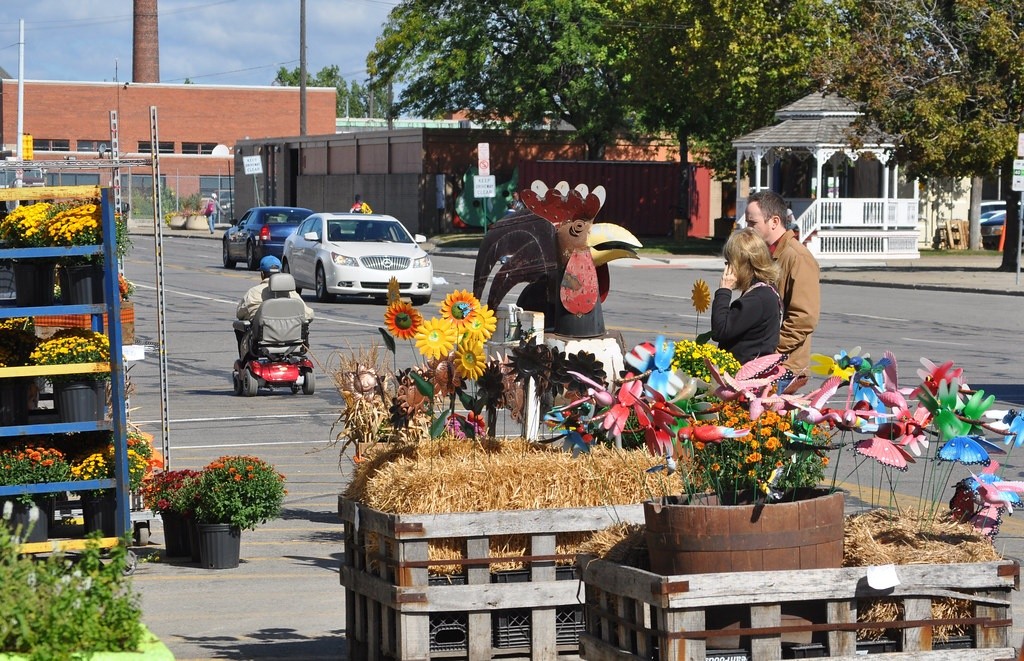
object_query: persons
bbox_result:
[350,194,373,215]
[206,192,226,235]
[710,227,780,366]
[744,190,820,396]
[234,256,314,360]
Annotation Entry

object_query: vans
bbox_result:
[980,200,1022,215]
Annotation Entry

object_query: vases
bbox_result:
[8,257,60,307]
[642,487,845,650]
[52,379,104,422]
[80,496,115,537]
[0,379,28,427]
[60,255,104,305]
[161,516,241,568]
[7,497,51,543]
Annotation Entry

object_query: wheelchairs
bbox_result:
[231,272,316,396]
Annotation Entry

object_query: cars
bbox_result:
[981,209,1024,250]
[281,211,434,306]
[222,206,322,270]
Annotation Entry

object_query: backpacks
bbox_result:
[353,208,363,213]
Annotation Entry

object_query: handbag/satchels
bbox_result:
[204,202,213,216]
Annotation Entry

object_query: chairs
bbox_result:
[286,214,302,223]
[329,224,342,239]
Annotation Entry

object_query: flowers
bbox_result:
[0,316,111,382]
[688,278,714,336]
[384,290,505,440]
[0,431,288,531]
[662,338,829,507]
[0,196,137,305]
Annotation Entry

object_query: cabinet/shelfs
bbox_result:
[0,188,136,575]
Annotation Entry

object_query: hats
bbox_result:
[211,193,217,199]
[257,255,282,272]
[355,194,360,199]
